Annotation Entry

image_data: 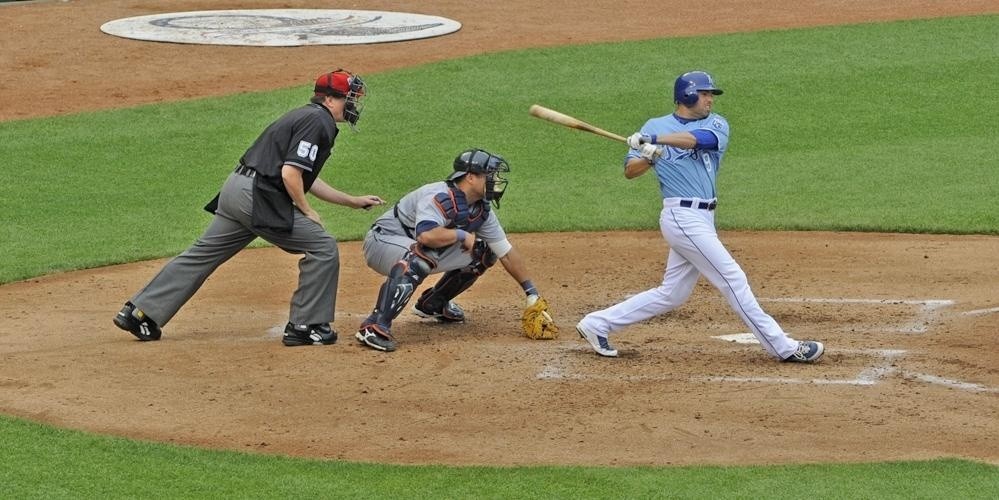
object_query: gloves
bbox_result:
[640,143,657,164]
[627,132,653,150]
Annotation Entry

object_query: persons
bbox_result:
[355,149,548,352]
[113,68,387,348]
[575,70,824,362]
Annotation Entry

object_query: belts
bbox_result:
[680,200,717,210]
[236,165,256,178]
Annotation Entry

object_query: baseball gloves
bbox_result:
[522,296,560,340]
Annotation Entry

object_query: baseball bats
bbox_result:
[529,104,664,157]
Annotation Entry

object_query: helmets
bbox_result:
[314,68,368,128]
[447,150,509,210]
[674,72,723,106]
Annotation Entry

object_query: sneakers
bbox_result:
[355,326,395,352]
[413,296,464,322]
[575,320,617,357]
[113,301,161,341]
[283,321,336,345]
[783,341,824,363]
